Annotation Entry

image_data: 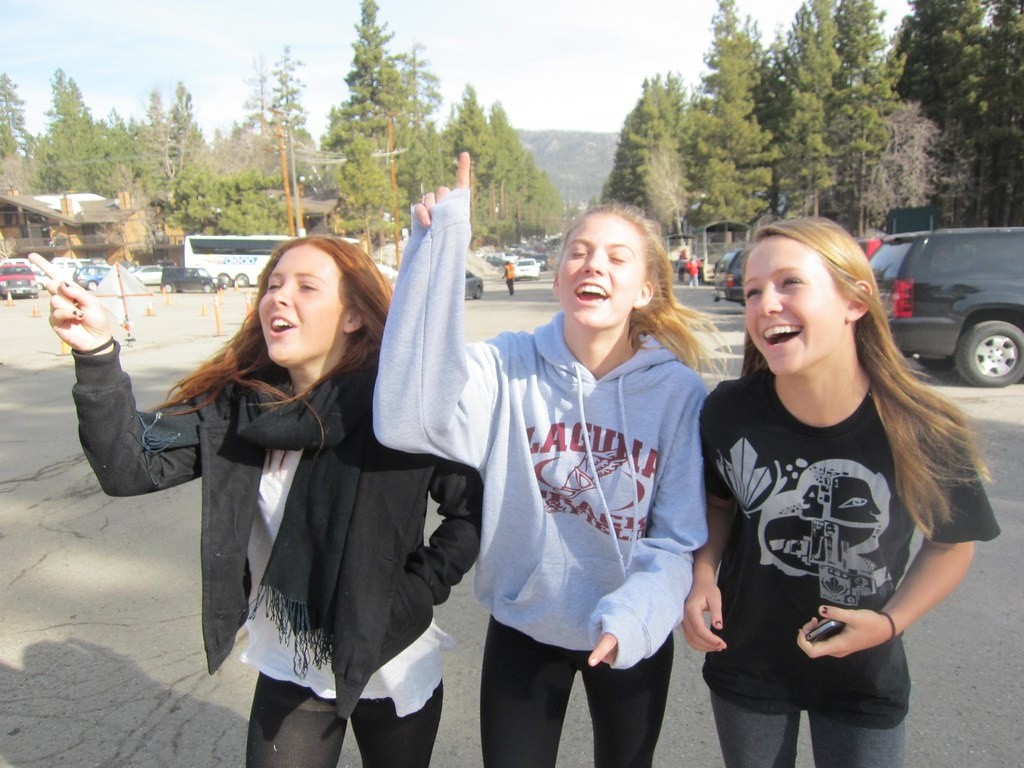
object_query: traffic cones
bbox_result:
[161,288,168,297]
[145,306,157,317]
[167,295,172,305]
[200,304,208,316]
[4,291,15,307]
[232,280,240,291]
[31,300,42,319]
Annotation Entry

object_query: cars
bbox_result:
[514,258,541,281]
[465,269,484,300]
[485,239,561,271]
[0,264,38,298]
[31,266,50,291]
[129,265,162,284]
[72,266,111,291]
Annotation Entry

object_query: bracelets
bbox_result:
[878,611,895,641]
[76,335,113,355]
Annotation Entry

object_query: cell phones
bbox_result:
[806,619,844,643]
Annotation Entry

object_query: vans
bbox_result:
[160,267,215,293]
[0,258,30,270]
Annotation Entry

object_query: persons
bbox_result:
[682,215,1002,768]
[676,255,685,282]
[685,256,703,288]
[713,256,722,273]
[502,261,514,295]
[372,152,709,768]
[28,236,485,768]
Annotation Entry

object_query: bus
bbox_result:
[184,235,360,288]
[691,220,751,284]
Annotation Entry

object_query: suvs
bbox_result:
[50,258,94,269]
[712,250,747,306]
[868,226,1023,388]
